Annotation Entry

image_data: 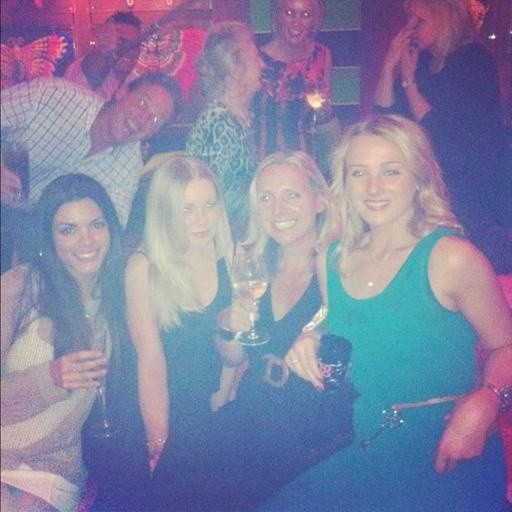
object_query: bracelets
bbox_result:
[146,438,166,447]
[485,381,512,413]
[399,79,415,88]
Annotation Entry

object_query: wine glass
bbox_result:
[235,253,271,345]
[306,78,324,124]
[74,316,121,439]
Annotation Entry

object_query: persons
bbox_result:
[148,146,360,512]
[61,13,141,99]
[184,20,265,245]
[0,174,123,512]
[373,0,512,276]
[244,0,332,182]
[255,114,512,512]
[0,72,183,274]
[81,152,237,512]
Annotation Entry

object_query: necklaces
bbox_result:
[350,231,406,290]
[78,297,100,322]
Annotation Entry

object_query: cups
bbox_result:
[316,334,351,394]
[3,146,28,202]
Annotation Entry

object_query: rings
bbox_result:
[68,361,78,373]
[290,359,298,365]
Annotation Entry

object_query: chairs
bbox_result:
[477,273,512,512]
